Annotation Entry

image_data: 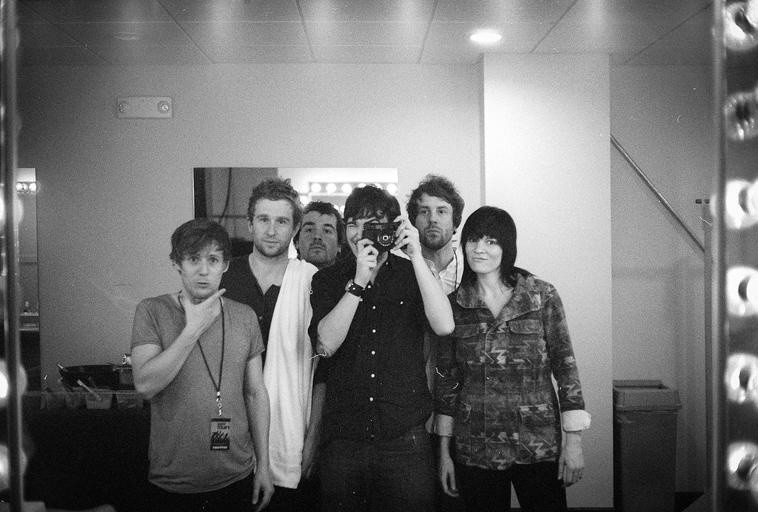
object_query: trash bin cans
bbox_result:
[612,379,682,512]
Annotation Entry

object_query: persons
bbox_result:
[406,173,467,511]
[129,215,277,510]
[308,184,458,511]
[292,199,344,272]
[216,177,331,512]
[433,205,592,512]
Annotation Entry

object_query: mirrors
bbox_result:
[193,167,399,259]
[4,3,724,511]
[15,165,43,392]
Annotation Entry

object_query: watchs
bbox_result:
[345,279,365,297]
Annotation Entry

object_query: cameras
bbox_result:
[363,219,400,251]
